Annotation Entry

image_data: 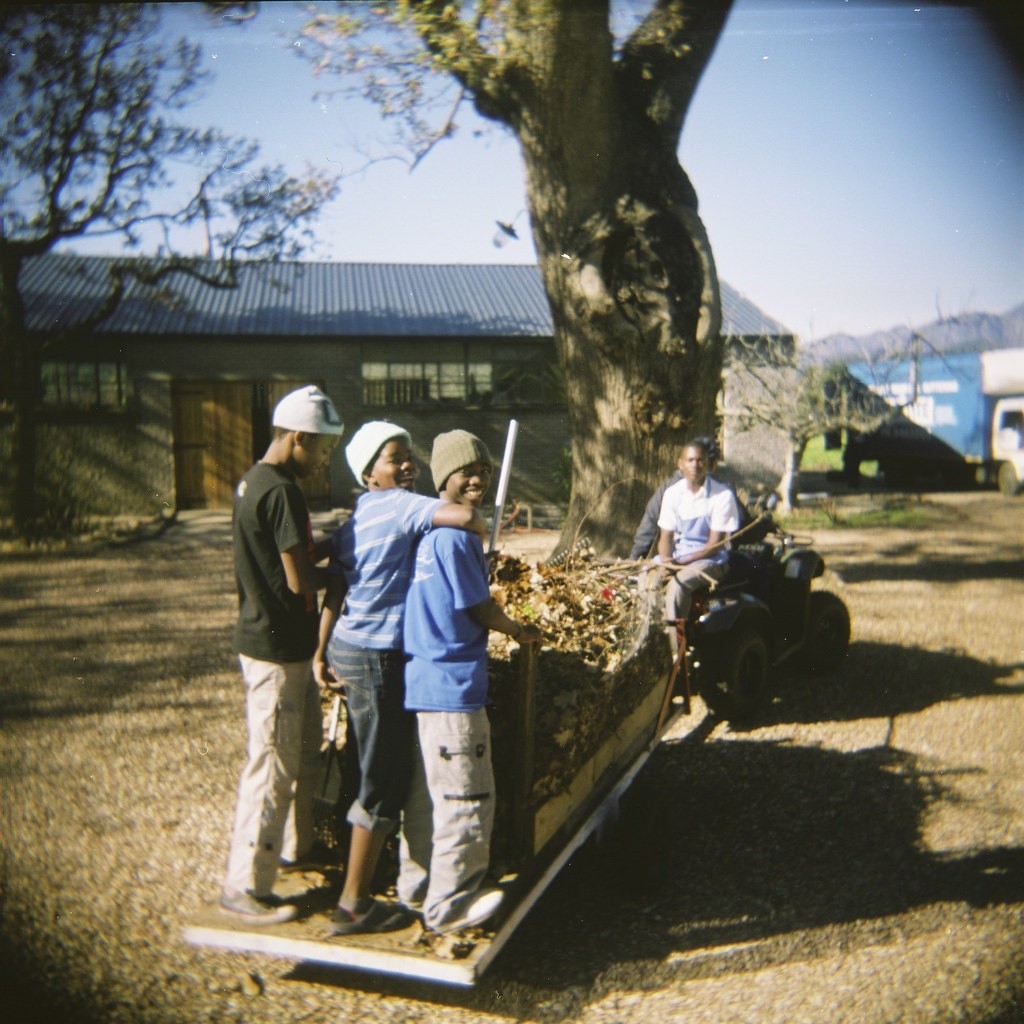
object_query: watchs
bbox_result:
[510,618,523,640]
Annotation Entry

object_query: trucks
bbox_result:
[840,351,1024,498]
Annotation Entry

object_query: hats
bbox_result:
[273,384,345,436]
[431,427,493,492]
[345,420,412,489]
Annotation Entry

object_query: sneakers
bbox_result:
[330,894,411,934]
[217,886,297,926]
[277,839,334,869]
[398,890,422,911]
[431,890,507,934]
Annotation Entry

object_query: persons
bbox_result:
[310,419,488,936]
[638,440,740,676]
[219,383,345,923]
[628,437,772,588]
[396,429,541,937]
